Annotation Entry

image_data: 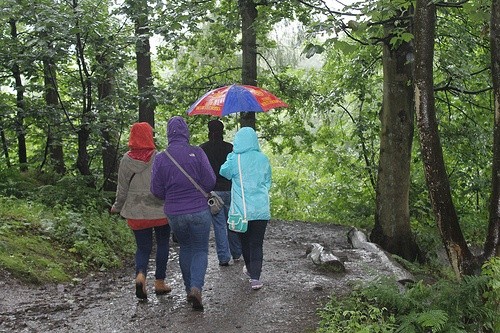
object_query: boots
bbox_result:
[154,279,171,294]
[135,273,148,299]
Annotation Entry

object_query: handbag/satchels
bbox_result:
[209,191,224,215]
[227,214,248,232]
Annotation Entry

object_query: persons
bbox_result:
[111,121,172,299]
[219,127,272,289]
[199,120,241,265]
[151,116,217,310]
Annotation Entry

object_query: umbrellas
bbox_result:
[186,85,289,130]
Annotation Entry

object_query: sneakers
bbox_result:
[243,265,251,278]
[249,279,263,289]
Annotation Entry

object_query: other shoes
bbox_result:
[234,257,240,261]
[219,261,228,266]
[187,288,203,309]
